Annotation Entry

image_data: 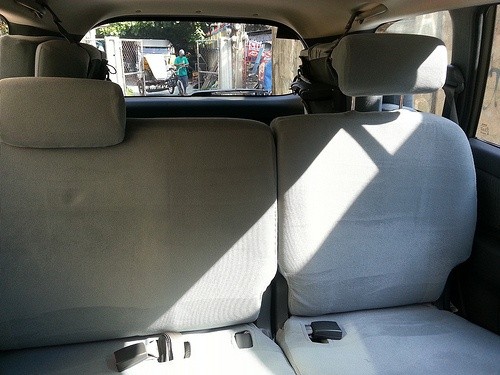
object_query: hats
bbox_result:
[179,49,185,55]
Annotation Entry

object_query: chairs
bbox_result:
[0,33,500,375]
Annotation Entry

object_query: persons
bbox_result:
[173,49,189,95]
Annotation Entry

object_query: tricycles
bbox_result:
[138,54,188,95]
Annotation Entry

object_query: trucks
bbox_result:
[134,39,175,78]
